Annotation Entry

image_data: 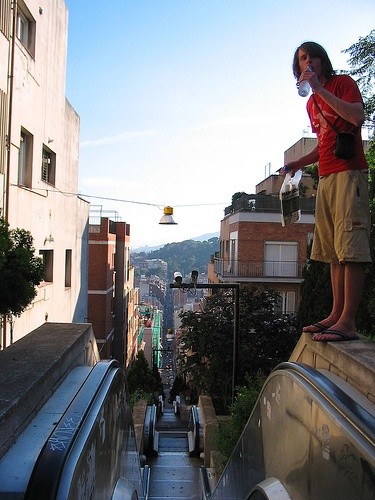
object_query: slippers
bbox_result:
[311,330,359,342]
[301,322,330,333]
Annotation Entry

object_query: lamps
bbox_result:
[158,207,178,226]
[173,270,199,295]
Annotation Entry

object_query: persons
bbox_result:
[278,42,372,343]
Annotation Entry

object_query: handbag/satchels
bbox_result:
[333,132,359,160]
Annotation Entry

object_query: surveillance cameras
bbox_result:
[190,271,198,284]
[173,271,183,283]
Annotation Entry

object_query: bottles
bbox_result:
[283,185,299,225]
[299,67,313,97]
